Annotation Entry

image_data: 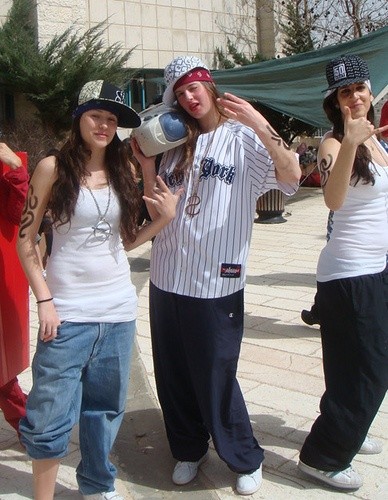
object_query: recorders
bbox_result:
[132,100,199,157]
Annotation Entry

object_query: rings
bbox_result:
[152,200,155,203]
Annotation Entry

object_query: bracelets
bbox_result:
[37,297,53,304]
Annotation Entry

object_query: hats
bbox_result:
[320,54,369,93]
[73,80,141,128]
[162,56,210,105]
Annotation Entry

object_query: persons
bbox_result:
[0,143,28,433]
[16,79,185,500]
[132,57,302,496]
[298,54,388,488]
[301,134,388,329]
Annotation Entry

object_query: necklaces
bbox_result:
[185,115,221,217]
[85,176,112,241]
[365,140,374,150]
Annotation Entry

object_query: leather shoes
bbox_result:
[301,310,320,325]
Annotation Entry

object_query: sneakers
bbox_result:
[83,490,122,500]
[298,461,364,489]
[357,436,382,454]
[235,462,262,494]
[172,450,209,485]
